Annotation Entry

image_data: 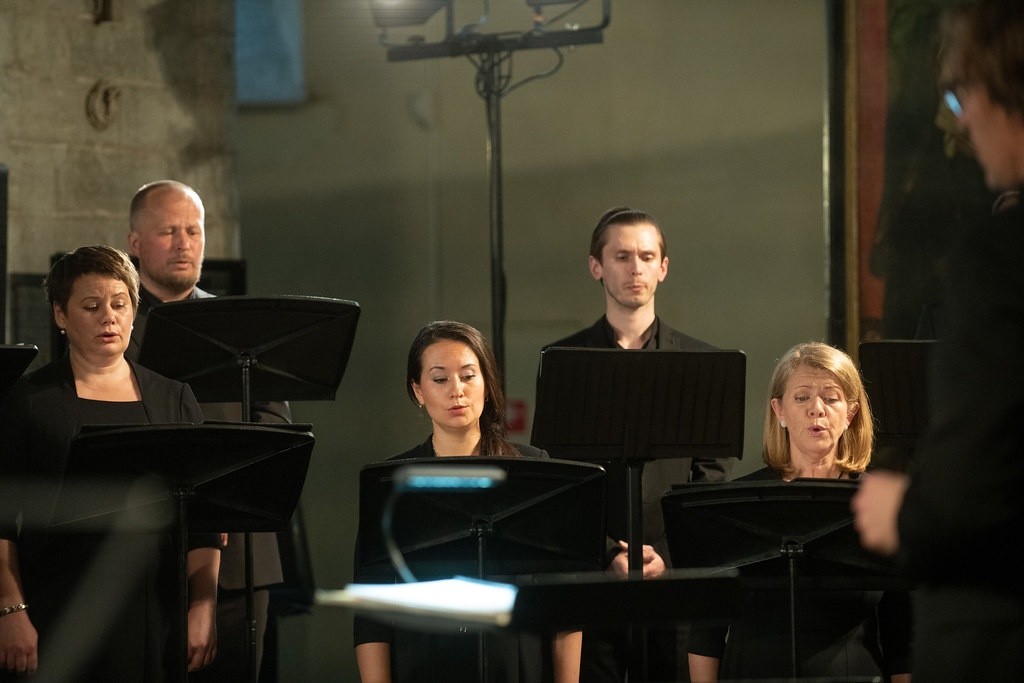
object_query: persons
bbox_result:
[847,0,1024,682]
[528,207,734,580]
[0,244,224,683]
[124,179,292,683]
[674,341,914,683]
[351,320,585,683]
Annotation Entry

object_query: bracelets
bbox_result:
[0,603,29,617]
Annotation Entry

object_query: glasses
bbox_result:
[920,84,967,119]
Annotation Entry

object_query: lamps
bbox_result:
[367,0,612,61]
